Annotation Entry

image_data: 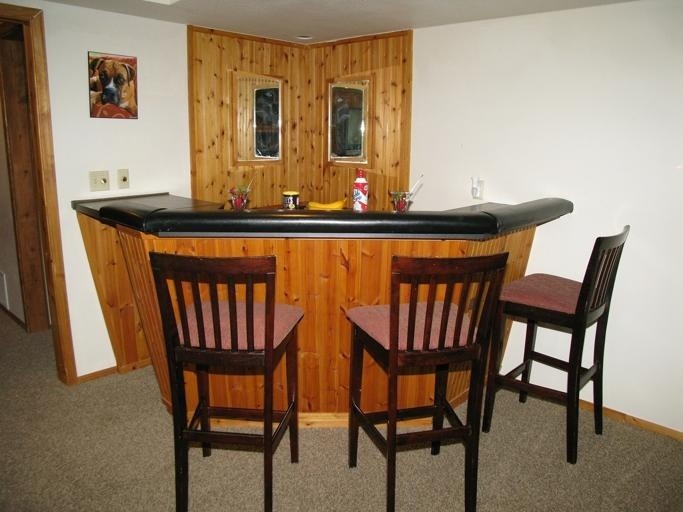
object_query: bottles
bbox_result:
[353,169,369,210]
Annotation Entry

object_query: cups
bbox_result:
[227,197,251,209]
[390,201,413,211]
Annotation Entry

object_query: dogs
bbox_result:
[90,58,138,117]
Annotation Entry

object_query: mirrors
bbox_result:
[323,74,375,170]
[229,69,286,166]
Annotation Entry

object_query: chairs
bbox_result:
[146,251,304,512]
